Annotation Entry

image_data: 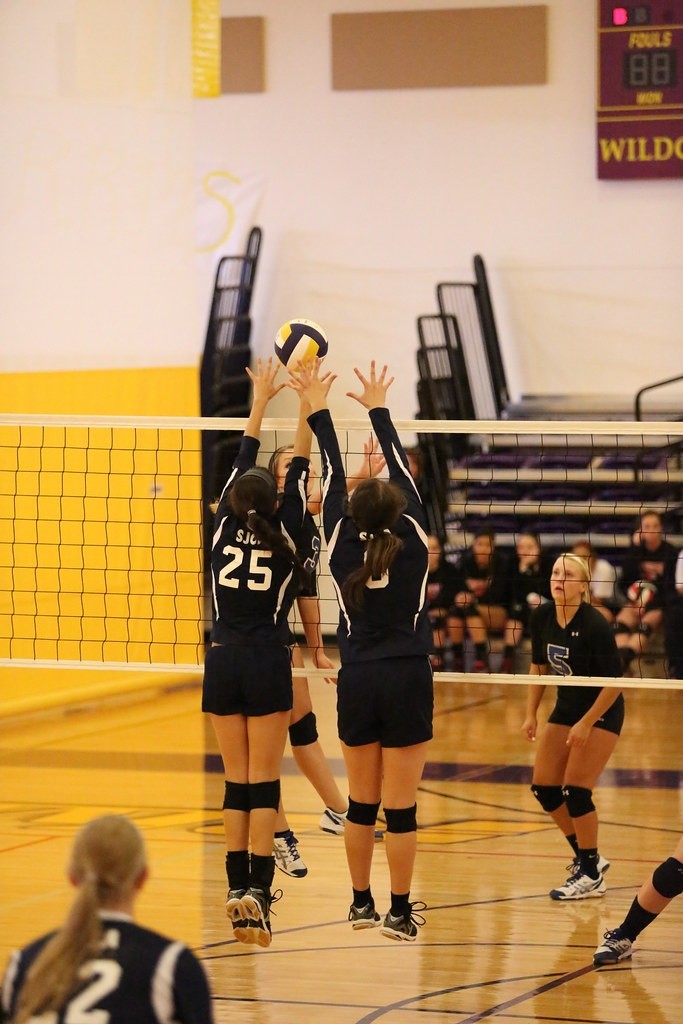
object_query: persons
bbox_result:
[593,833,683,963]
[0,815,213,1024]
[402,440,683,677]
[268,340,385,877]
[202,358,332,945]
[524,554,626,900]
[292,359,433,942]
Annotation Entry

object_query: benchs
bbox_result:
[445,392,683,577]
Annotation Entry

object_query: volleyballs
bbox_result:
[274,318,329,370]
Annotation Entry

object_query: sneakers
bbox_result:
[225,887,283,947]
[321,808,384,843]
[380,900,426,941]
[347,903,383,931]
[548,873,608,900]
[565,850,611,874]
[593,927,632,963]
[272,831,308,878]
